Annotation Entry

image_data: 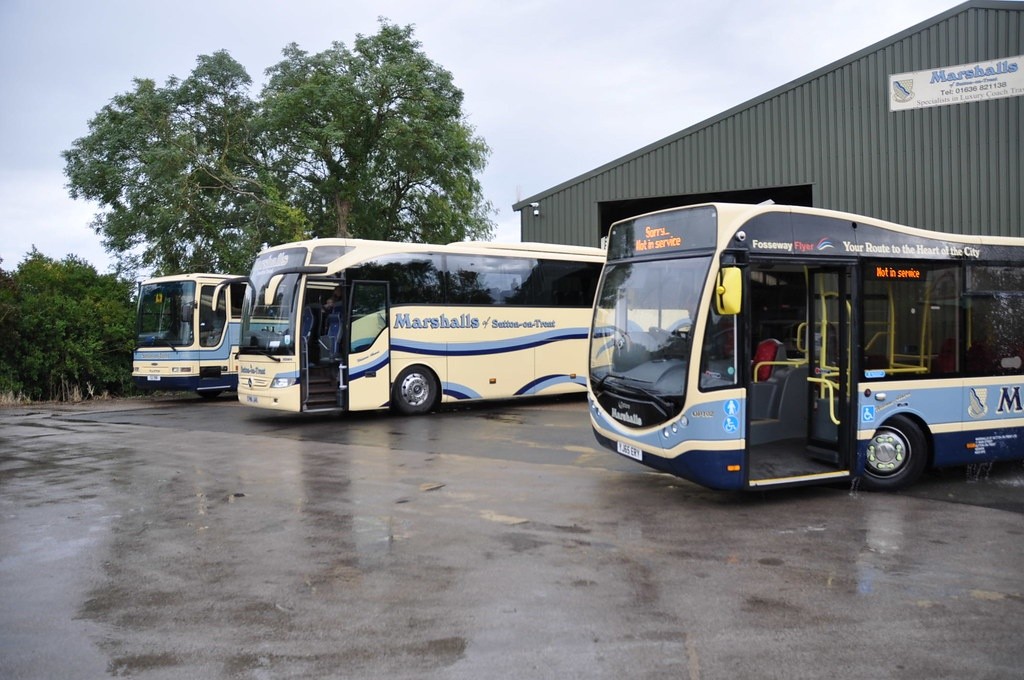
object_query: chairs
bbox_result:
[752,338,786,378]
[327,302,343,353]
[932,337,971,376]
[302,306,314,345]
[969,339,996,376]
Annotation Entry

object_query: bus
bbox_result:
[237,236,692,420]
[133,274,249,400]
[584,200,1023,498]
